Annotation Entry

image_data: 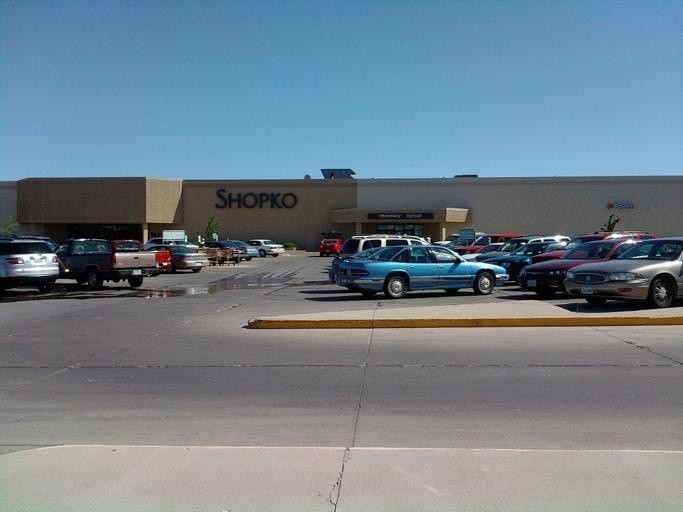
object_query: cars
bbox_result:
[0,235,285,294]
[333,244,510,299]
[562,236,683,308]
[319,238,344,256]
[515,238,640,297]
[329,231,656,283]
